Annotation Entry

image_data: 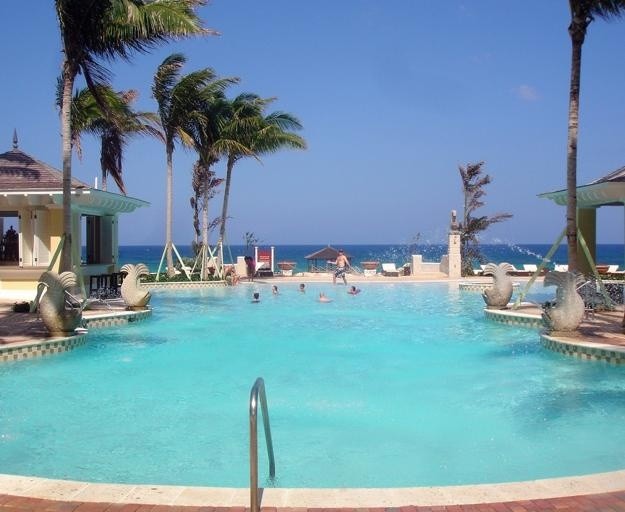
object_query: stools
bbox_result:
[89,272,125,297]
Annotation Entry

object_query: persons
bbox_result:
[347,285,361,294]
[326,248,355,287]
[298,283,306,295]
[271,285,277,295]
[250,292,261,304]
[318,292,330,301]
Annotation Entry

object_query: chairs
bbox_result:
[381,262,399,278]
[238,261,265,279]
[478,262,625,276]
